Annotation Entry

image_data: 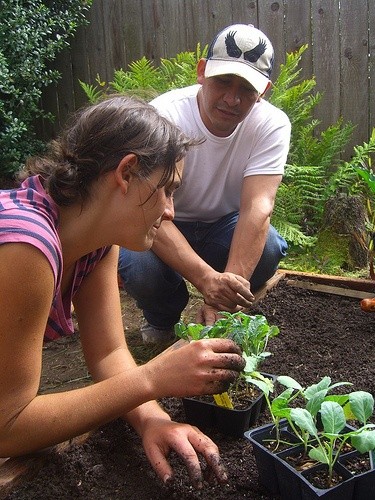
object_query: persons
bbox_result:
[117,23,292,351]
[0,90,246,492]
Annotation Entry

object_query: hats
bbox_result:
[205,23,275,94]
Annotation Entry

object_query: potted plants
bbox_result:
[174,309,281,439]
[244,375,375,500]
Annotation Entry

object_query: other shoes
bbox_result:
[139,310,175,347]
